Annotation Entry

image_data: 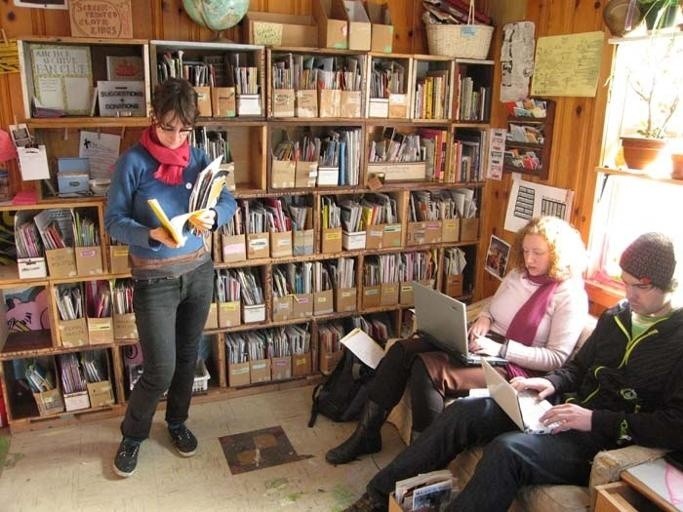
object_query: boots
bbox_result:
[325,397,390,465]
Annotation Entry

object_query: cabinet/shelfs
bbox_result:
[0,37,496,436]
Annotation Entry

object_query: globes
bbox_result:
[183,0,249,43]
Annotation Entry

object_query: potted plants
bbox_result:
[618,74,681,170]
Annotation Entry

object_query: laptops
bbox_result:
[480,357,570,435]
[412,280,509,366]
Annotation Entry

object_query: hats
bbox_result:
[619,232,677,291]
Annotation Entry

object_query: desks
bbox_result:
[593,459,683,512]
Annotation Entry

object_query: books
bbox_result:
[148,52,492,124]
[16,46,146,416]
[190,127,494,384]
[393,469,461,510]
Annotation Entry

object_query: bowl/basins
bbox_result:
[88,177,112,197]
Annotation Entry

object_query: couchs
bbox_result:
[385,280,672,512]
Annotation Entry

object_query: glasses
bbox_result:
[155,114,193,136]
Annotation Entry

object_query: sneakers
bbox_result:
[338,493,386,512]
[167,424,199,457]
[112,436,141,477]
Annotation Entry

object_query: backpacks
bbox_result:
[306,338,384,429]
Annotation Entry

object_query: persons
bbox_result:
[341,231,683,512]
[104,78,239,478]
[326,214,591,466]
